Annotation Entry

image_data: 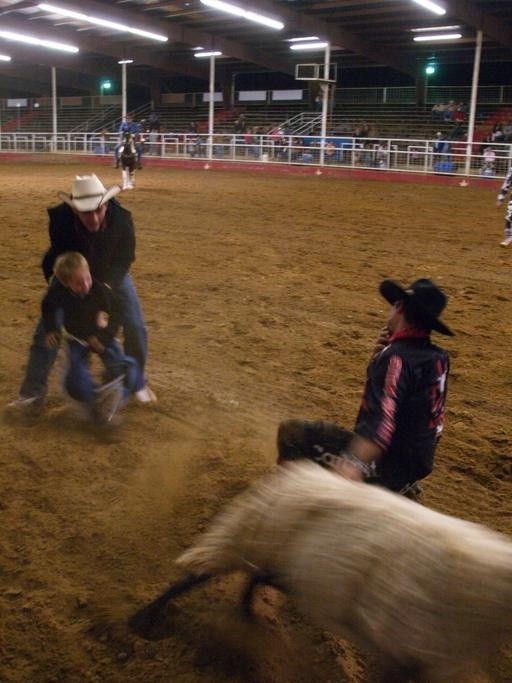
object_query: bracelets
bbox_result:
[338,448,373,478]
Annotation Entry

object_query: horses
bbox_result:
[120,126,138,191]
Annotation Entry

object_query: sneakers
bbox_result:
[114,160,143,170]
[93,384,122,427]
[5,396,47,413]
[135,383,157,407]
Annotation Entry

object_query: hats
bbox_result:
[378,277,456,337]
[57,173,121,213]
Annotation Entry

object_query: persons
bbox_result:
[6,172,158,414]
[432,99,468,121]
[352,120,388,167]
[479,122,512,176]
[99,127,109,153]
[146,111,223,156]
[234,112,335,163]
[277,279,456,504]
[435,132,451,153]
[433,156,452,172]
[114,115,143,169]
[41,252,139,425]
[496,175,512,246]
[451,123,464,138]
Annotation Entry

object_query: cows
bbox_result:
[126,458,512,683]
